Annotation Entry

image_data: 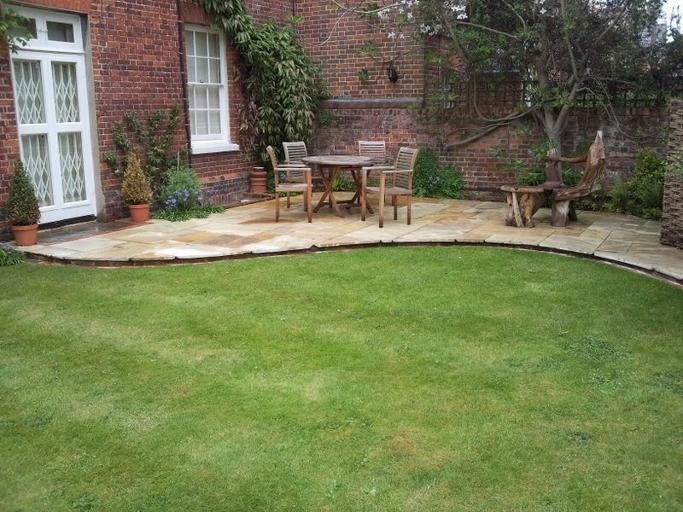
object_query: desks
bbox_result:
[301,154,374,219]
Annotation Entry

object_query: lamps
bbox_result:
[386,63,397,83]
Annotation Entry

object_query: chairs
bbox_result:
[266,144,314,224]
[281,140,332,213]
[357,139,395,206]
[359,146,419,229]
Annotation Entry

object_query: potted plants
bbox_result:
[3,155,42,248]
[120,140,153,223]
